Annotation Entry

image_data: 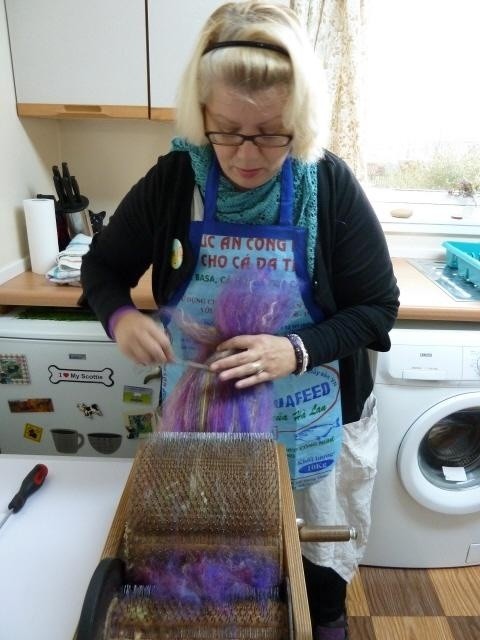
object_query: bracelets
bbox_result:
[284,334,303,377]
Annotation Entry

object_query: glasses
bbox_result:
[201,107,294,148]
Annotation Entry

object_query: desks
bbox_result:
[0,452,136,638]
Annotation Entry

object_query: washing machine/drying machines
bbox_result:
[357,319,473,570]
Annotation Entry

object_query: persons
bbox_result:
[80,0,403,640]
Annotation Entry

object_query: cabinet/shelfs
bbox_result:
[3,0,292,122]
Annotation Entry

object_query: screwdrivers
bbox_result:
[0,464,48,528]
